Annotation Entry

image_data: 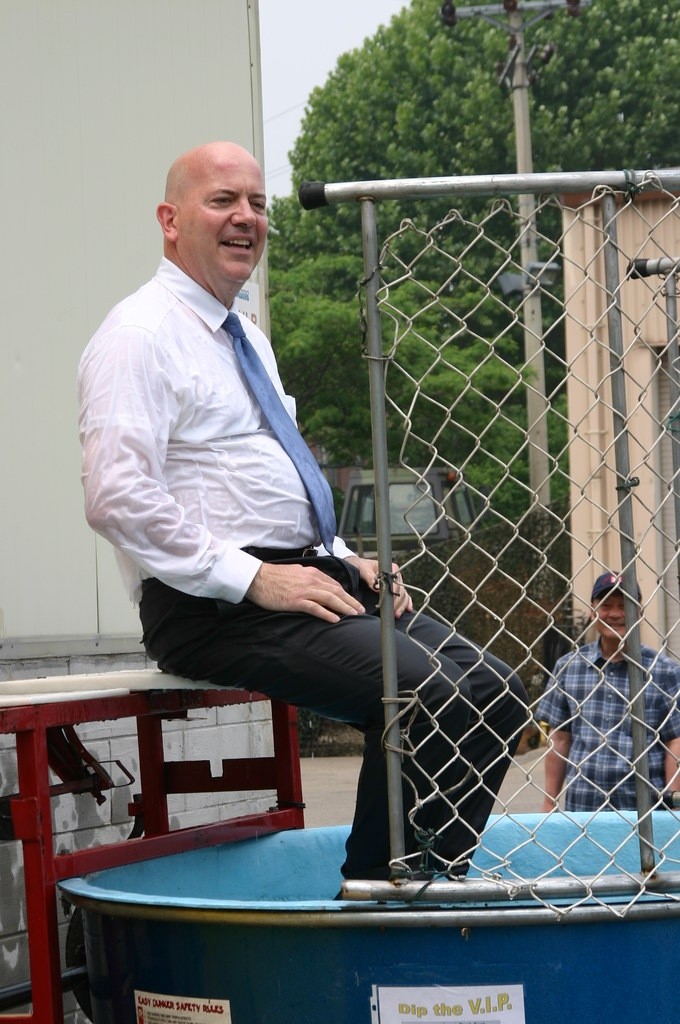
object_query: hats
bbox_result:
[591,570,642,603]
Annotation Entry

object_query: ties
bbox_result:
[222,311,337,555]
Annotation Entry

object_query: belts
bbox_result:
[242,545,321,562]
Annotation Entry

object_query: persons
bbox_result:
[535,570,680,813]
[79,142,528,899]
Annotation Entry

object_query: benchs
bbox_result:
[0,682,313,1022]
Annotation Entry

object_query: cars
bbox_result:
[336,470,478,553]
